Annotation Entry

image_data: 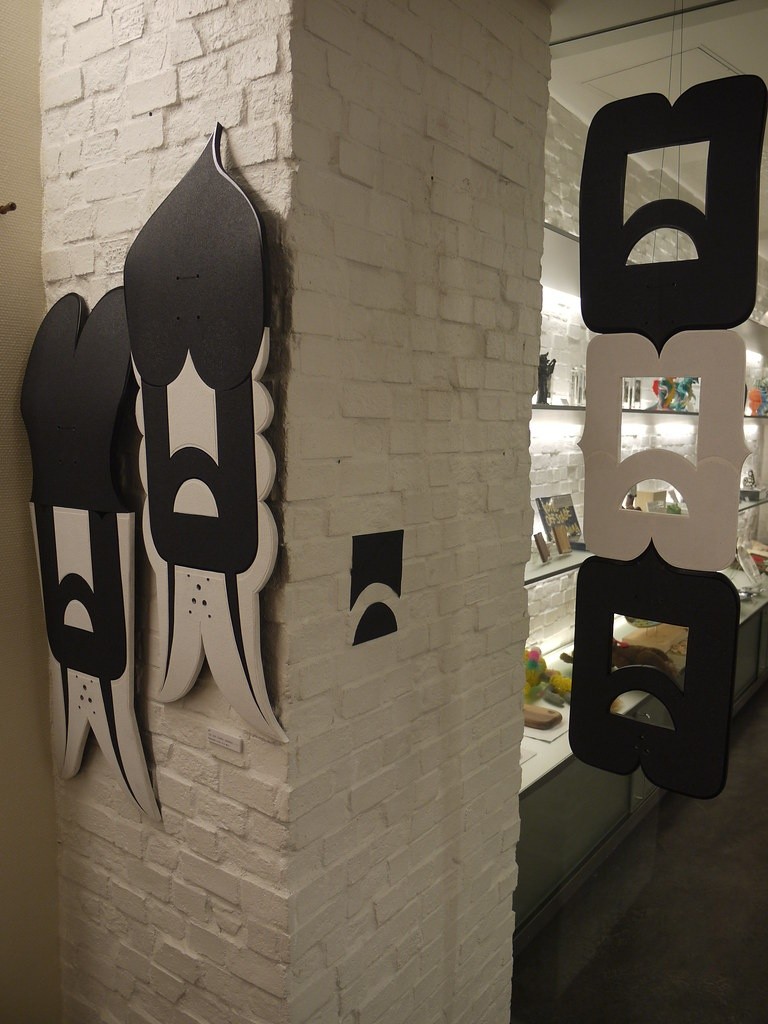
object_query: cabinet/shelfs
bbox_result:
[513,206,768,962]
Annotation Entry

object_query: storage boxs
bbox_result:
[740,487,766,501]
